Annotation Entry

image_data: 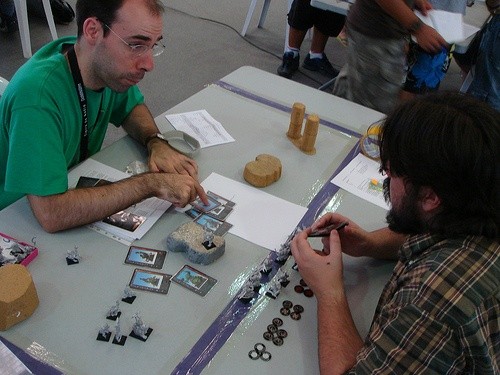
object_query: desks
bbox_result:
[1,62,394,375]
[309,0,479,92]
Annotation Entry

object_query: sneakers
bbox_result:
[303,51,339,79]
[277,49,300,79]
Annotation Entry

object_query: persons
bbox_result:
[0,0,210,234]
[277,0,500,116]
[289,89,500,375]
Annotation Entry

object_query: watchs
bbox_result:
[145,132,168,147]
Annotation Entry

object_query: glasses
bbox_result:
[106,22,166,57]
